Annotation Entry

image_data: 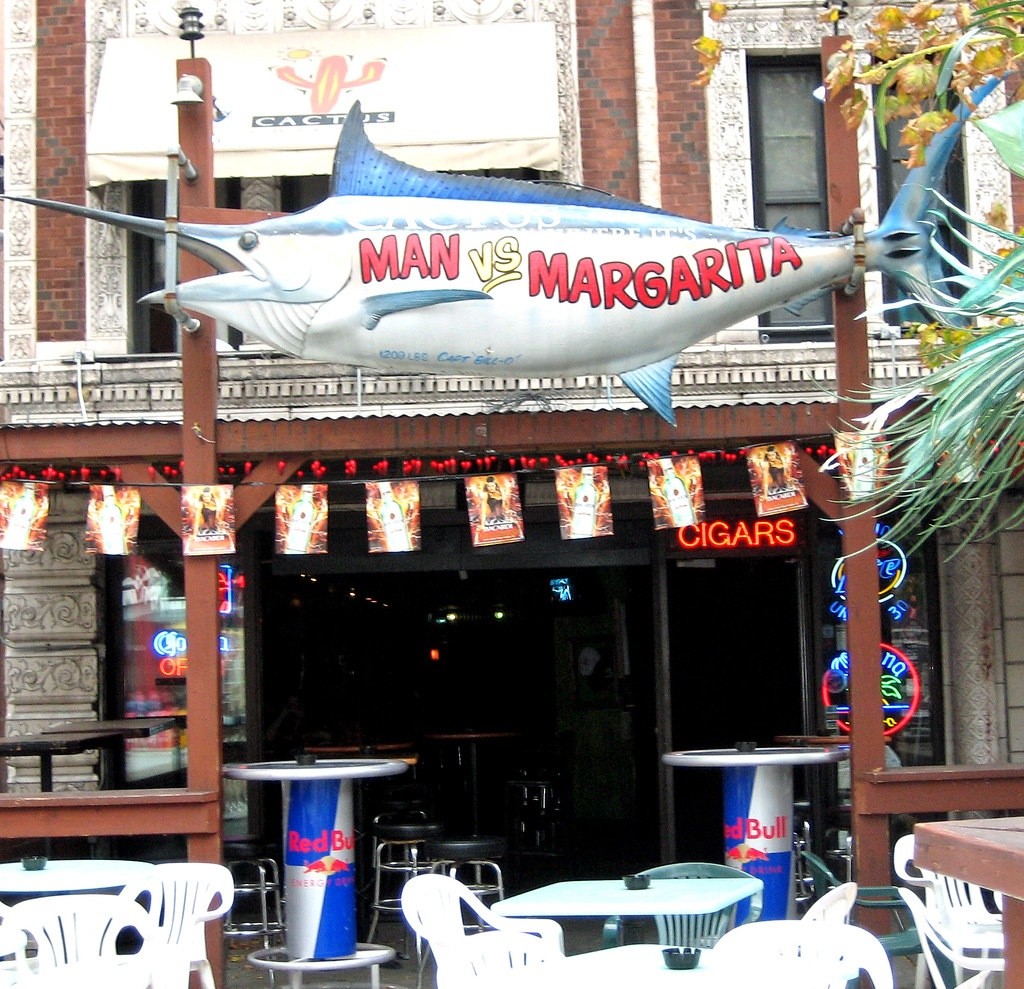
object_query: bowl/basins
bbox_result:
[21,855,47,870]
[295,754,317,765]
[623,874,650,889]
[663,947,701,970]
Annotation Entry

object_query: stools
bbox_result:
[793,800,850,911]
[223,839,286,988]
[365,818,447,973]
[505,765,563,857]
[416,833,504,989]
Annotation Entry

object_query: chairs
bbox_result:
[893,835,1005,989]
[0,862,895,989]
[801,850,958,989]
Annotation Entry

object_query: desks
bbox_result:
[490,878,764,950]
[0,859,153,964]
[774,734,819,831]
[42,717,176,792]
[426,729,520,837]
[662,746,851,930]
[459,944,712,989]
[303,737,415,836]
[220,758,408,989]
[0,733,124,793]
[804,736,893,859]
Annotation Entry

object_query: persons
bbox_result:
[765,447,787,492]
[200,487,217,530]
[484,477,504,518]
[552,579,571,601]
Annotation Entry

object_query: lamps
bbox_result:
[177,7,204,60]
